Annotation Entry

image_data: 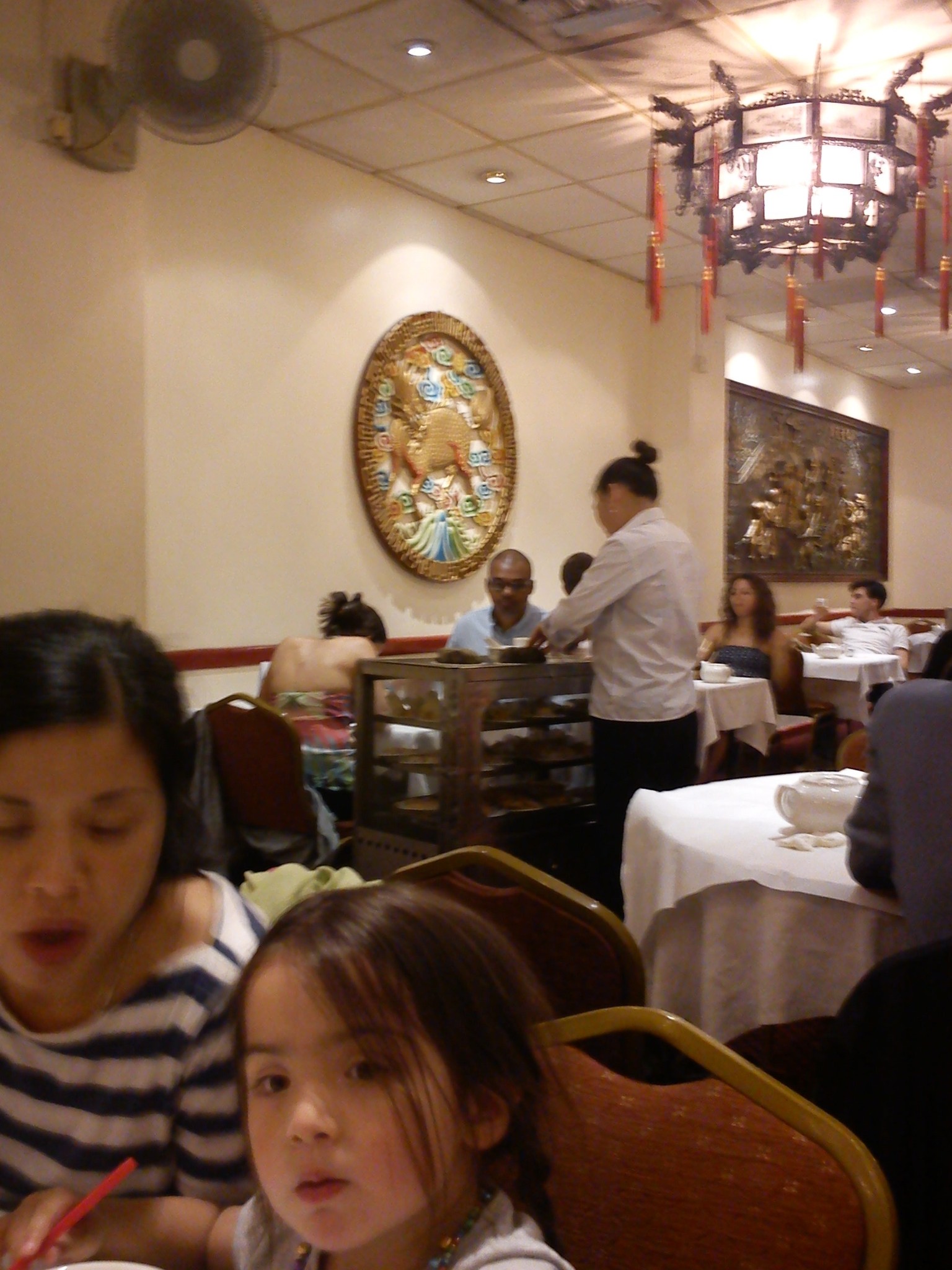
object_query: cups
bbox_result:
[513,636,529,647]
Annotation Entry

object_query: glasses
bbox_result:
[487,578,533,596]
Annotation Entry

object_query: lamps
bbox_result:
[647,61,952,371]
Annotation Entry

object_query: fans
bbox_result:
[53,0,278,174]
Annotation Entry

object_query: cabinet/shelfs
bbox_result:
[356,658,595,875]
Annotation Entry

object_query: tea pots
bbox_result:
[774,773,869,832]
[699,660,730,683]
[810,643,845,658]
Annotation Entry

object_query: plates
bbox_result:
[485,645,544,663]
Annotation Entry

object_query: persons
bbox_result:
[445,550,549,655]
[0,609,271,1205]
[526,438,701,923]
[798,579,909,771]
[560,552,594,595]
[255,590,429,819]
[691,573,794,785]
[0,881,577,1270]
[844,630,952,947]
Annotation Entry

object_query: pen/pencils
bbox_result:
[10,1158,137,1270]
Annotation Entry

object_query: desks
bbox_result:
[621,632,940,1048]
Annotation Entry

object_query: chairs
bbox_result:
[392,843,892,1270]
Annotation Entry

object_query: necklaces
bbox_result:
[291,1188,496,1270]
